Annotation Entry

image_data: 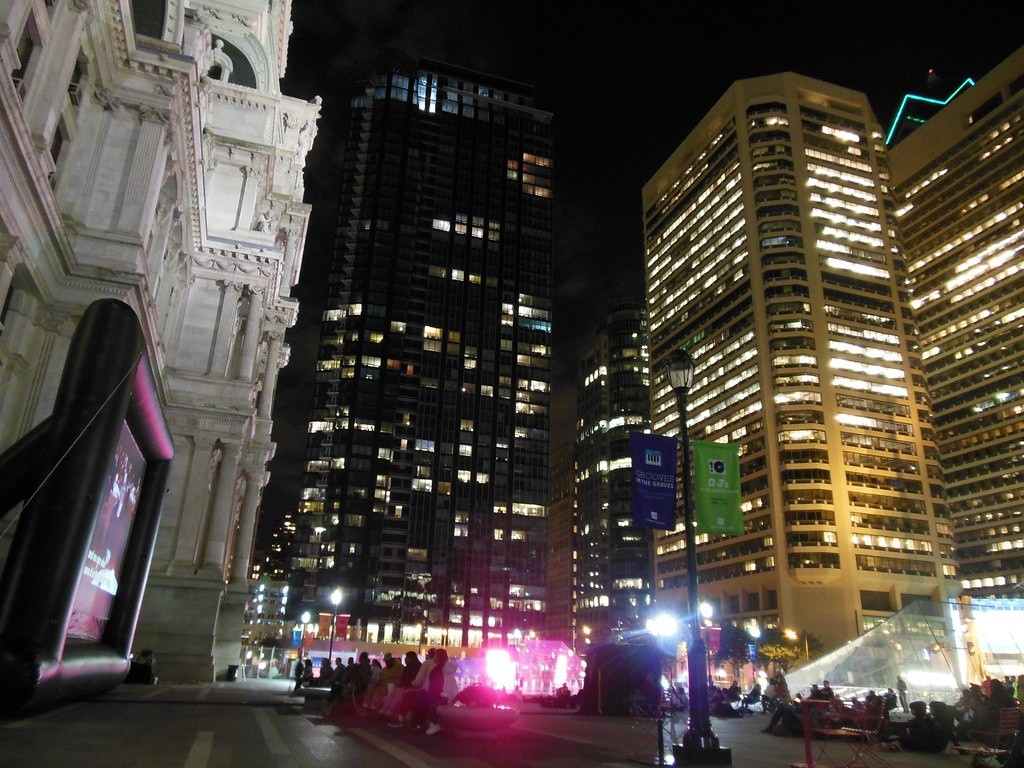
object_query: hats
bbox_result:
[303,659,311,663]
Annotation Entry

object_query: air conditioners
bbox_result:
[12,77,27,105]
[68,82,84,106]
[48,174,57,191]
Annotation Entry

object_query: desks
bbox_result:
[951,745,1007,768]
[811,727,860,768]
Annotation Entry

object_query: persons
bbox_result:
[649,670,1024,755]
[293,647,460,737]
[494,681,586,709]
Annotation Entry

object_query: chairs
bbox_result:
[842,696,893,768]
[967,706,1020,768]
[625,667,678,753]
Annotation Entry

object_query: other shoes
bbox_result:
[425,722,441,735]
[388,722,405,728]
[760,726,772,734]
